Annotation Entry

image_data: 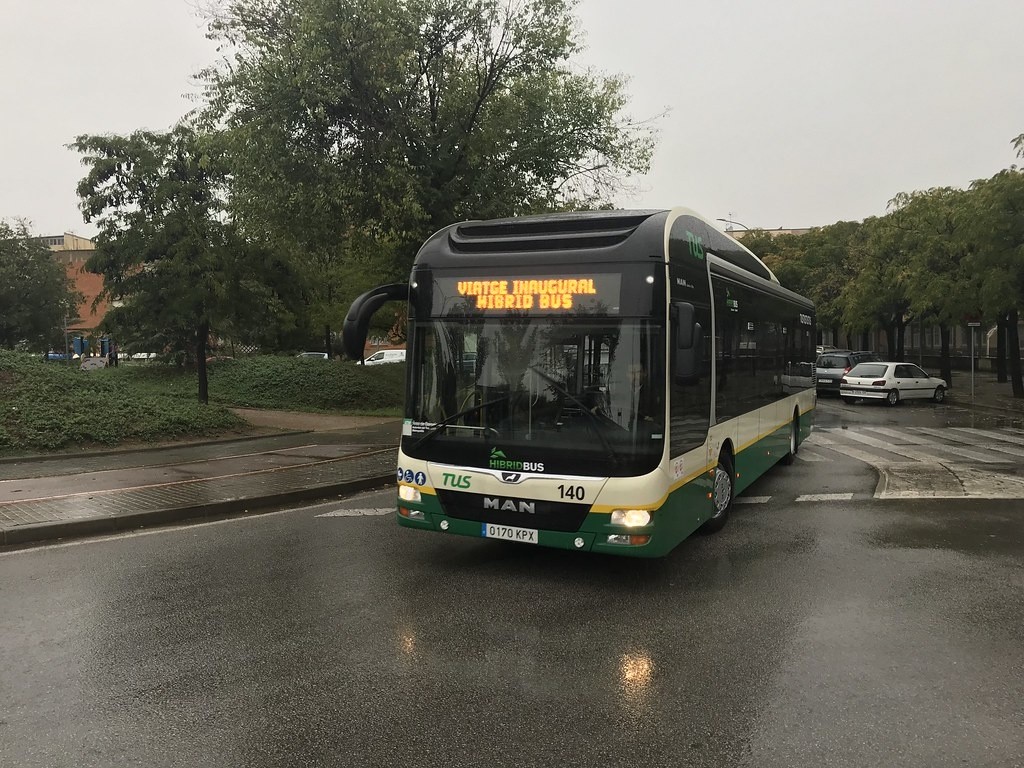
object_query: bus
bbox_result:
[341,207,816,561]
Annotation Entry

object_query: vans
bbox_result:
[356,349,406,367]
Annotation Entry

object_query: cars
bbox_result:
[455,352,477,374]
[773,361,814,395]
[839,362,947,407]
[206,355,234,363]
[297,352,328,360]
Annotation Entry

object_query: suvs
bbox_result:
[816,344,837,358]
[816,348,889,397]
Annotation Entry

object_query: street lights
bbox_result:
[968,322,981,400]
